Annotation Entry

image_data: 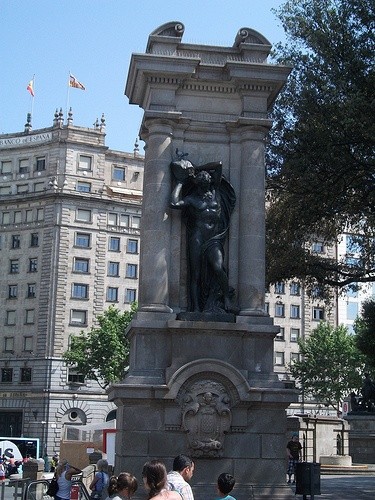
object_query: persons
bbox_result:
[0,454,34,479]
[189,392,230,442]
[213,472,236,500]
[54,457,81,500]
[287,431,302,485]
[106,472,138,500]
[90,459,111,500]
[143,460,183,500]
[168,160,236,311]
[167,454,195,500]
[43,454,58,472]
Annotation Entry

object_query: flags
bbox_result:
[69,74,85,90]
[26,80,34,96]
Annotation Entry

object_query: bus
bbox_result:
[0,437,40,478]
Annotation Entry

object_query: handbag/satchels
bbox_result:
[90,489,103,500]
[47,480,59,496]
